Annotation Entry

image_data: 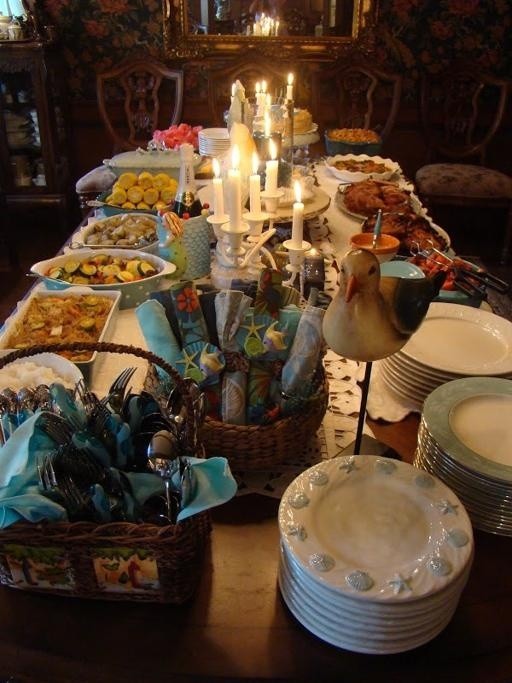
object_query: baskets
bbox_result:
[139,345,331,470]
[0,342,212,605]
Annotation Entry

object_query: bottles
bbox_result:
[166,145,211,280]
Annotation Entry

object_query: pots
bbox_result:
[7,114,33,147]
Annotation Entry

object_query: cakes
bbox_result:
[283,109,313,134]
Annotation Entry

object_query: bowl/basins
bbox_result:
[0,252,177,405]
[324,127,401,178]
[103,148,202,175]
[294,110,320,147]
[61,177,178,253]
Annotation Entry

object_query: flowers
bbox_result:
[34,0,162,81]
[377,0,512,72]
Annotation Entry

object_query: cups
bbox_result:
[0,16,23,41]
[1,81,29,105]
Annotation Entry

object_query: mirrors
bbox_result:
[162,0,379,61]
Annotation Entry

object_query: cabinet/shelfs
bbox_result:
[0,40,69,209]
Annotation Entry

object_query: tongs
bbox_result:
[409,239,510,300]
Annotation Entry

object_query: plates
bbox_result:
[384,299,509,409]
[198,127,230,154]
[277,453,475,657]
[337,179,452,280]
[412,374,509,534]
[195,177,328,224]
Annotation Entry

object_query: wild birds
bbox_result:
[322,248,448,362]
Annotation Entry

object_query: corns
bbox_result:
[105,171,179,210]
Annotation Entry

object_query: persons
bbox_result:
[249,0,289,36]
